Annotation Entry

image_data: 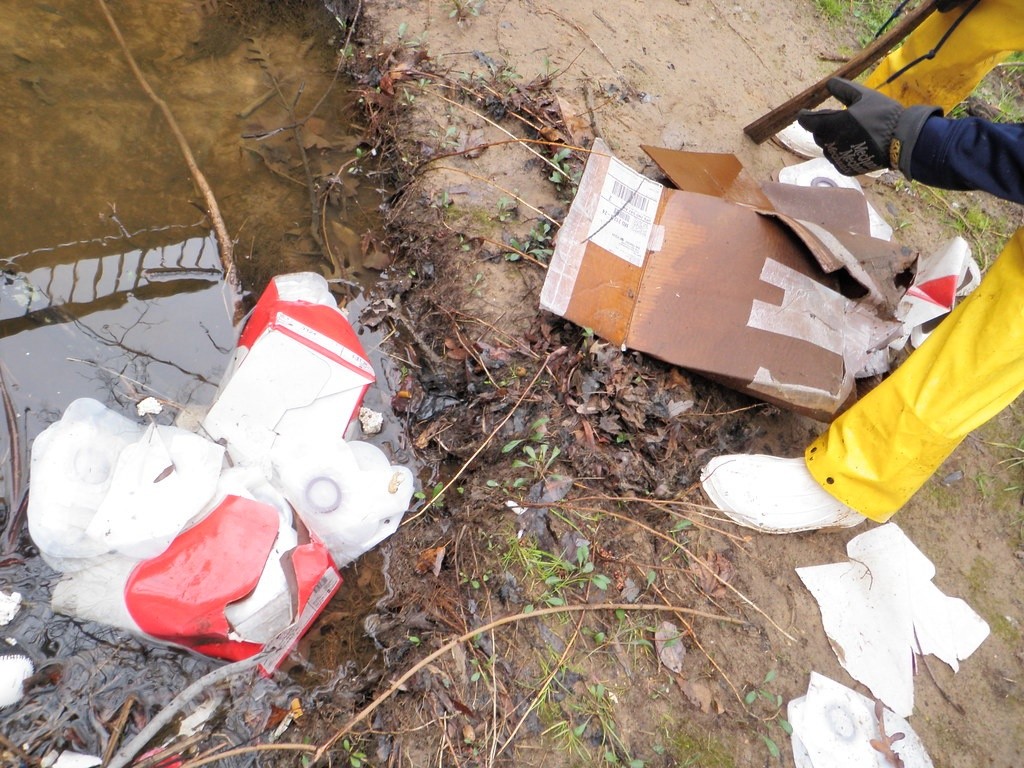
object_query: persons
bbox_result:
[699,1,1024,535]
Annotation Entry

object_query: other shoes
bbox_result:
[771,121,824,159]
[700,454,868,535]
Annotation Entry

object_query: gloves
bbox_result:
[796,77,944,182]
